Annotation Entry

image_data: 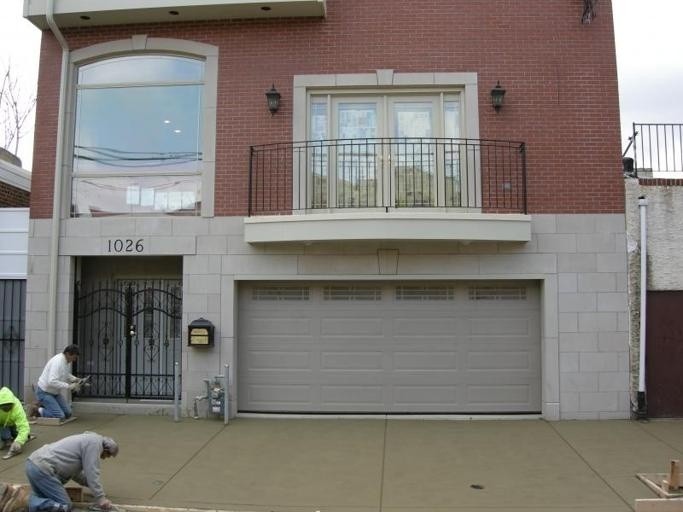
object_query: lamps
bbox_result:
[489,80,506,113]
[264,80,281,116]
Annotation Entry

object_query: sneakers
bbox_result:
[26,401,39,416]
[0,483,24,512]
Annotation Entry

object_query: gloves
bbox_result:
[9,443,21,453]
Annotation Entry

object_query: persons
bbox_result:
[0,430,120,512]
[27,343,92,423]
[0,387,31,455]
